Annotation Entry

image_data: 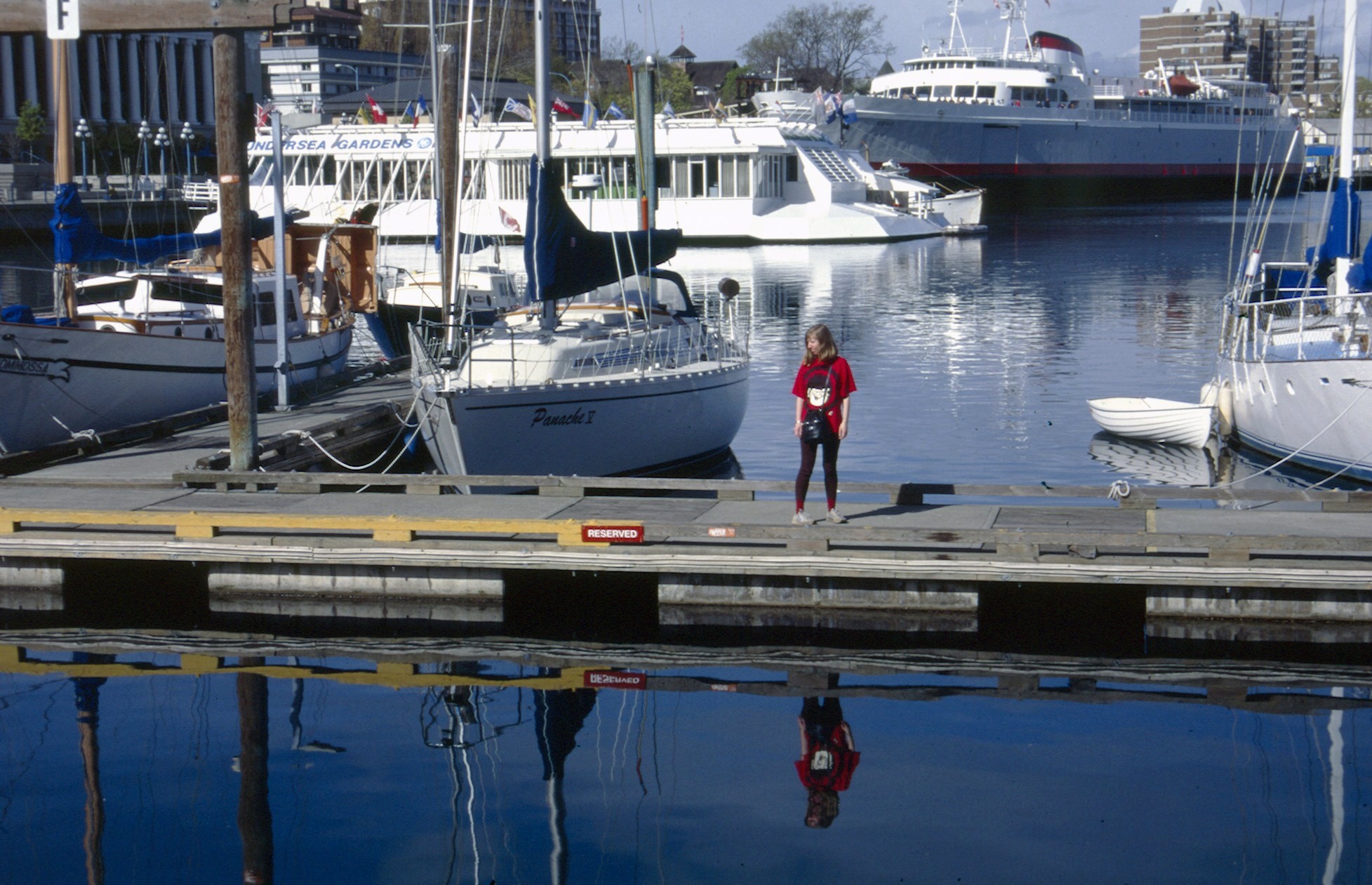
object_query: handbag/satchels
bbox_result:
[804,710,835,745]
[800,411,832,444]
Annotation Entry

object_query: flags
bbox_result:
[664,103,674,118]
[357,94,387,124]
[256,99,273,127]
[608,101,626,120]
[582,92,598,130]
[552,97,581,118]
[406,95,426,129]
[499,206,521,233]
[808,87,858,126]
[504,96,531,121]
[468,93,482,128]
[528,94,537,132]
[703,92,728,124]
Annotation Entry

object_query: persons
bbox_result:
[791,323,858,525]
[793,672,861,828]
[903,92,1075,108]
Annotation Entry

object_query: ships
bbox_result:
[751,0,1307,198]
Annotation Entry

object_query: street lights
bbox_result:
[154,128,170,174]
[334,63,361,92]
[693,86,718,104]
[74,118,92,190]
[138,120,153,176]
[180,122,196,183]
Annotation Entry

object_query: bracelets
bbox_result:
[796,421,802,423]
[800,730,806,734]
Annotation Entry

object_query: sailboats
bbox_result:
[1199,0,1372,485]
[408,1,751,496]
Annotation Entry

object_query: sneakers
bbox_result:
[826,507,847,523]
[791,510,814,525]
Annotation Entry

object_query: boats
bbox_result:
[1085,396,1215,449]
[1086,432,1215,490]
[192,1,989,244]
[1161,76,1201,99]
[1,111,380,475]
